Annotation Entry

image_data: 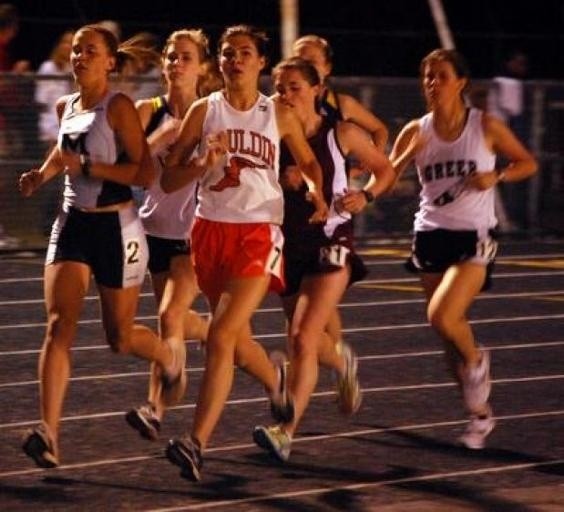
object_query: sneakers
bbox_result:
[125,338,203,482]
[22,422,59,468]
[456,413,494,450]
[463,347,491,411]
[255,344,362,463]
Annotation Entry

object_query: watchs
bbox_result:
[391,48,539,451]
[495,169,507,184]
[80,153,93,176]
[361,189,374,205]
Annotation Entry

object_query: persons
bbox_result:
[2,7,28,73]
[267,33,388,360]
[17,26,187,468]
[36,28,167,152]
[160,25,329,480]
[127,30,208,441]
[253,57,398,465]
